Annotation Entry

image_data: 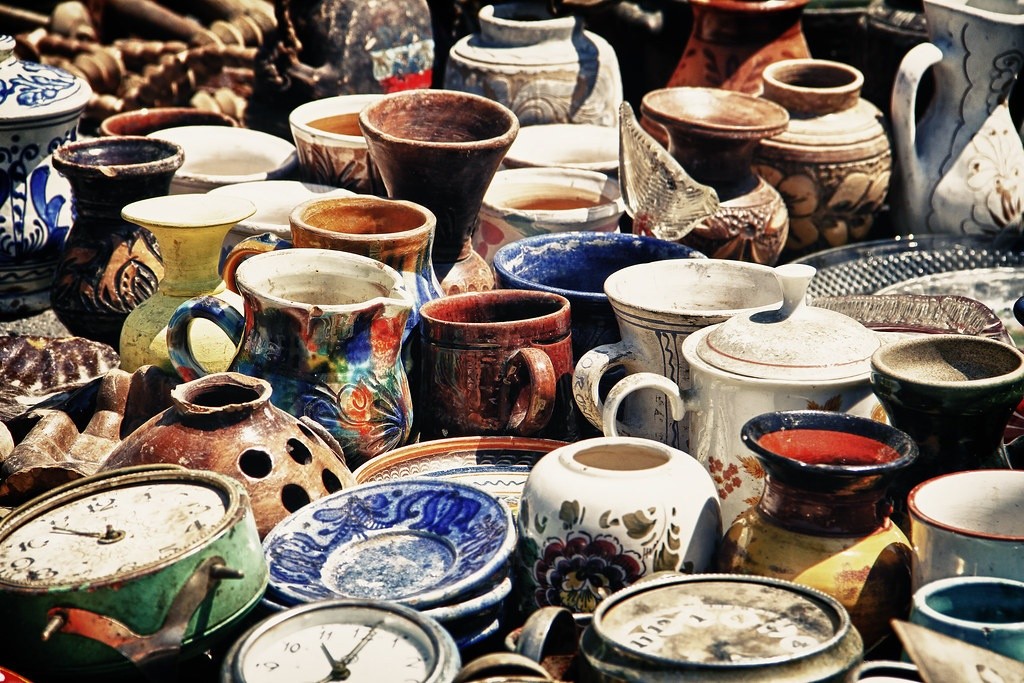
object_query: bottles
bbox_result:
[0,0,1024,682]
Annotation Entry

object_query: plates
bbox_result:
[790,234,1024,347]
